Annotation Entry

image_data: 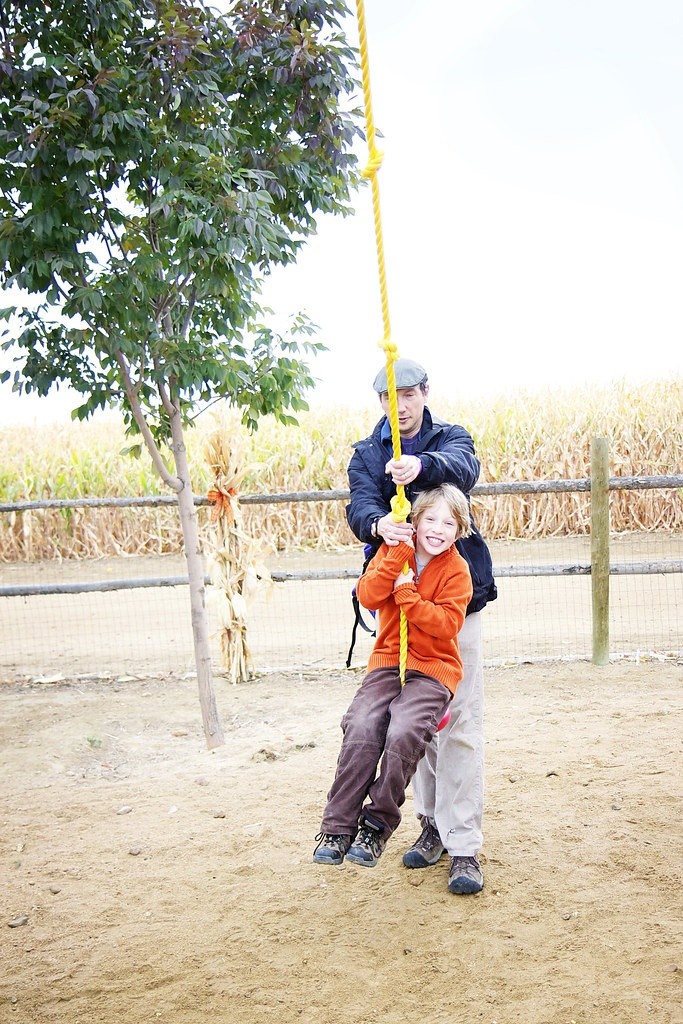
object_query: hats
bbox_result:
[373,358,428,393]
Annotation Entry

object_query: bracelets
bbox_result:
[374,516,379,540]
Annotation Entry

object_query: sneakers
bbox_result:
[403,813,445,868]
[344,814,392,867]
[448,854,483,894]
[312,833,357,865]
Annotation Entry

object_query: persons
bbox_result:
[345,359,497,894]
[312,482,477,868]
